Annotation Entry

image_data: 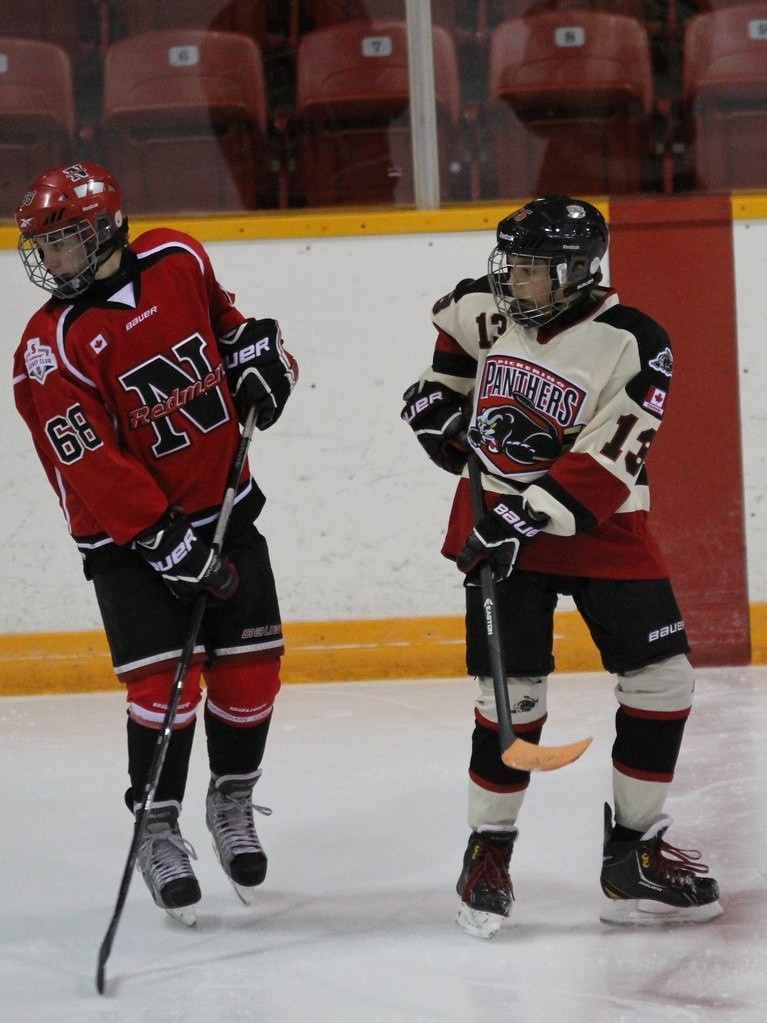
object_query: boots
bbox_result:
[455,824,518,938]
[599,802,722,929]
[133,769,267,925]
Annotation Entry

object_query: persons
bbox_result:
[12,158,303,926]
[397,182,729,938]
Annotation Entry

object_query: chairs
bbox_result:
[295,17,460,206]
[490,8,654,199]
[102,29,266,213]
[682,4,767,189]
[0,38,80,218]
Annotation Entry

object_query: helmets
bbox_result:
[487,196,611,330]
[14,161,128,301]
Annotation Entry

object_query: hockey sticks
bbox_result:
[97,402,258,994]
[465,451,594,772]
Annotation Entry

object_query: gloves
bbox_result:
[136,505,241,608]
[398,381,477,476]
[218,318,298,430]
[456,495,549,590]
